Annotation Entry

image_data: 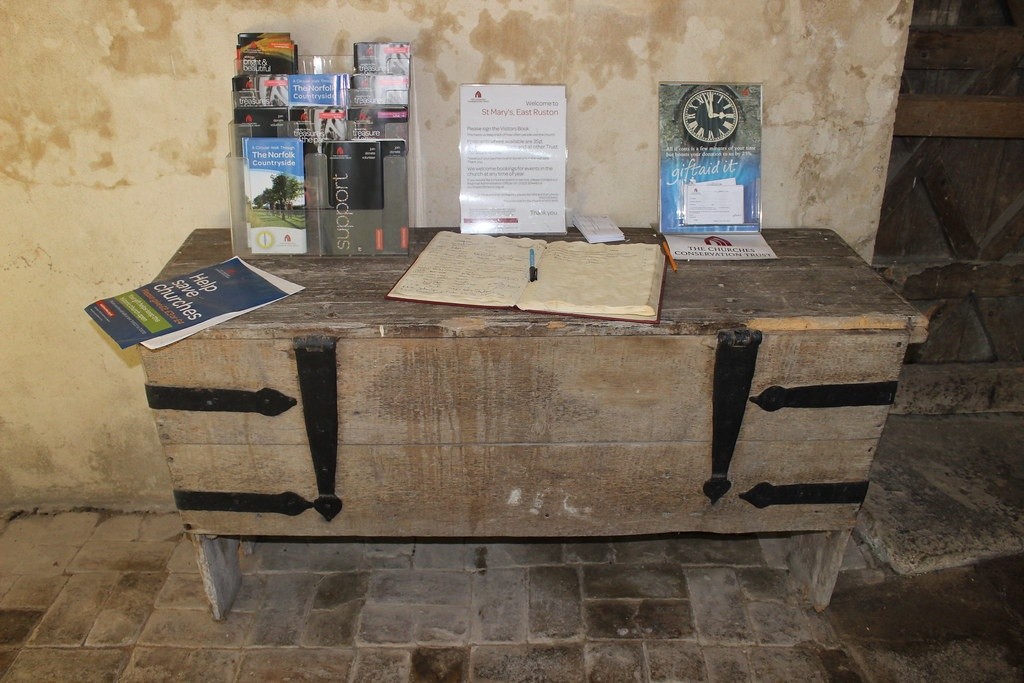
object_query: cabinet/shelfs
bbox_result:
[137,229,929,622]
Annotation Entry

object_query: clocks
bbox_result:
[682,90,738,142]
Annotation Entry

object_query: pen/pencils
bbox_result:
[663,241,678,273]
[530,248,538,282]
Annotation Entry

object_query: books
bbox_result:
[384,229,668,325]
